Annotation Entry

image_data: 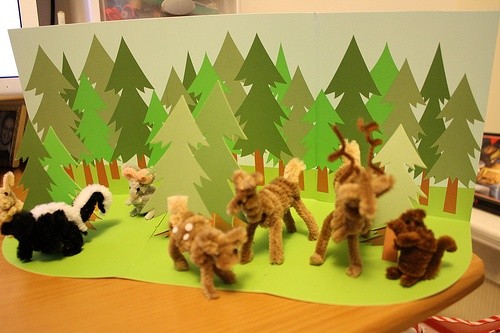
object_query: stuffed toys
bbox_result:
[310,122,396,276]
[166,197,247,299]
[0,171,113,262]
[123,168,159,219]
[228,157,320,266]
[387,209,458,287]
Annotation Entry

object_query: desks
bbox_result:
[1,170,486,333]
